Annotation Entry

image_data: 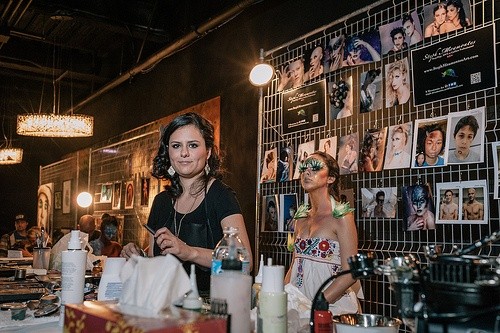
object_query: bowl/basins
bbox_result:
[332,314,402,333]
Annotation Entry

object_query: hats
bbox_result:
[14,213,28,222]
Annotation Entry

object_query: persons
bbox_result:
[323,124,410,218]
[387,61,410,106]
[449,115,480,163]
[440,189,458,220]
[407,184,434,230]
[330,81,352,119]
[463,188,483,220]
[79,214,122,258]
[8,215,53,256]
[278,47,323,91]
[284,151,359,315]
[388,15,421,53]
[415,127,446,167]
[261,148,309,231]
[324,35,380,74]
[119,112,253,305]
[425,0,470,37]
[361,69,382,113]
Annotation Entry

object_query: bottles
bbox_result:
[314,293,333,333]
[61,230,88,304]
[253,254,264,306]
[258,265,287,333]
[210,226,250,276]
[210,243,252,333]
[183,265,202,313]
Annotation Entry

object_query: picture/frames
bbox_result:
[54,191,63,210]
[61,178,71,214]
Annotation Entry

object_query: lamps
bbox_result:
[0,70,24,165]
[16,15,93,137]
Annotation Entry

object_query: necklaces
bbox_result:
[174,195,198,238]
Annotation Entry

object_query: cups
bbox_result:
[10,305,27,321]
[33,247,51,270]
